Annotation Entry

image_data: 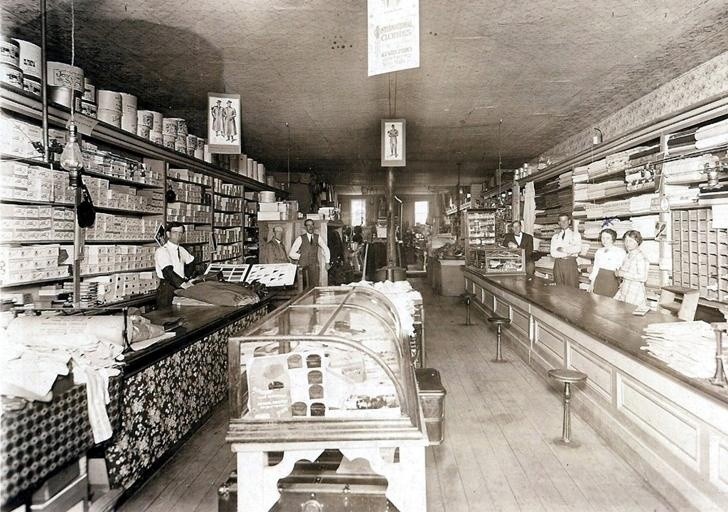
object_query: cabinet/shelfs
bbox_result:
[445,90,728,313]
[0,80,290,315]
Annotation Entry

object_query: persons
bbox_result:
[210,101,227,136]
[549,214,583,288]
[611,232,650,307]
[388,125,400,157]
[588,228,625,296]
[288,219,331,288]
[501,220,536,275]
[262,226,290,264]
[153,221,198,306]
[223,100,237,141]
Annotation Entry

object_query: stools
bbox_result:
[547,369,588,448]
[487,317,511,363]
[460,293,477,327]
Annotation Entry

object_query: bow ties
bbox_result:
[514,234,520,236]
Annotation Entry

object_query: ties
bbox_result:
[311,236,312,244]
[178,247,180,261]
[562,231,565,240]
